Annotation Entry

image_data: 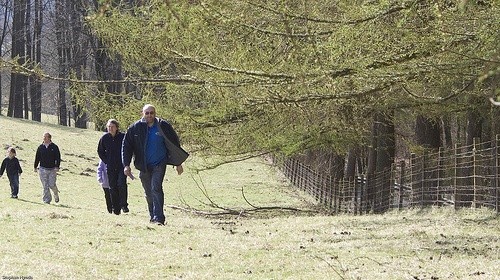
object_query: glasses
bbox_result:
[143,112,155,115]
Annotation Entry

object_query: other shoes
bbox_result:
[10,194,18,199]
[55,197,59,202]
[121,206,128,212]
[150,216,164,225]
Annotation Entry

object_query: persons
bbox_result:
[97,118,130,215]
[97,158,113,214]
[121,105,189,226]
[34,132,61,203]
[0,147,24,198]
